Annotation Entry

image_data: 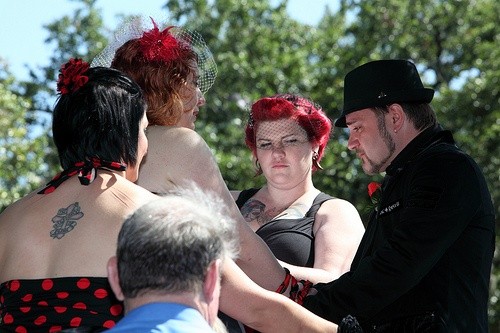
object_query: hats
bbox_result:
[334,60,434,128]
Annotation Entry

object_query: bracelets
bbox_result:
[271,266,315,306]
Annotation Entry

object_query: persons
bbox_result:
[111,34,318,299]
[225,91,367,283]
[301,61,496,333]
[1,65,340,333]
[101,196,224,333]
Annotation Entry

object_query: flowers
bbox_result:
[362,182,384,215]
[57,56,91,95]
